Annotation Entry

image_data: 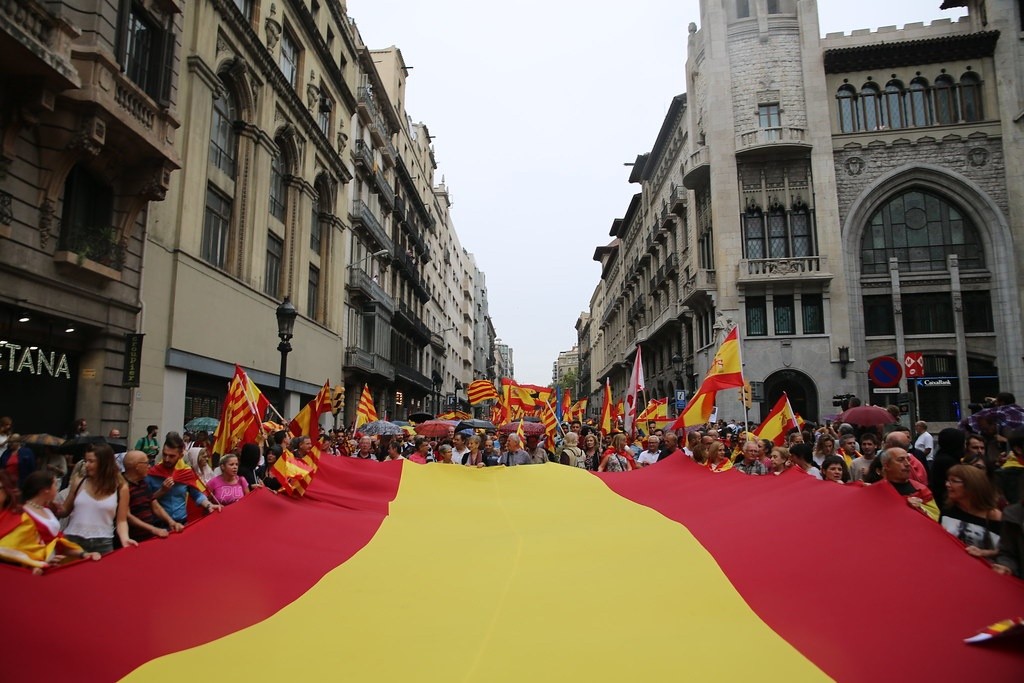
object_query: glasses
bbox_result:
[944,477,963,487]
[137,459,151,466]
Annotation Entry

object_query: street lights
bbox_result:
[671,353,684,417]
[276,297,299,424]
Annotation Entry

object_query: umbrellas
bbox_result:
[822,414,839,421]
[500,421,546,434]
[401,426,417,435]
[836,405,896,427]
[57,436,128,453]
[359,420,404,435]
[450,421,474,436]
[513,416,541,422]
[804,420,816,429]
[959,404,1024,436]
[414,420,456,437]
[455,418,496,433]
[8,433,67,446]
[392,420,411,426]
[662,420,704,436]
[408,411,436,422]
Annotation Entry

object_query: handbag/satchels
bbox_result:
[58,511,70,530]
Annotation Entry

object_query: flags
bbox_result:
[0,454,1024,683]
[213,301,804,498]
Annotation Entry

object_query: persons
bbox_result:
[185,416,219,431]
[0,391,1024,580]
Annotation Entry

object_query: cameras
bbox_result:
[968,401,995,411]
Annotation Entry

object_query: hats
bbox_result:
[563,432,579,447]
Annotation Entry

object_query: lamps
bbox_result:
[432,327,453,336]
[837,345,850,379]
[346,249,389,270]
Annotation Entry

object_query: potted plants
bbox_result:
[69,225,113,268]
